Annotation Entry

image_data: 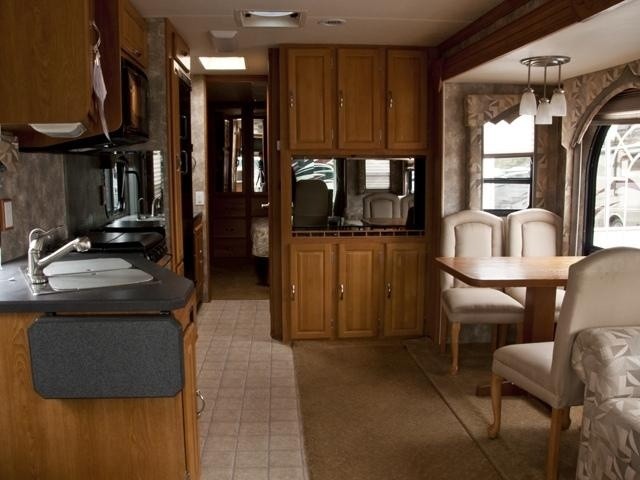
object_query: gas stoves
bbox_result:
[49,231,168,263]
[102,219,167,237]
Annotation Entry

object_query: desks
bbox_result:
[361,218,406,228]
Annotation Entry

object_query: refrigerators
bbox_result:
[179,77,195,286]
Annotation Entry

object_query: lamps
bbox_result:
[520,55,570,125]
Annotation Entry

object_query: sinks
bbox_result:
[43,257,133,277]
[44,267,156,291]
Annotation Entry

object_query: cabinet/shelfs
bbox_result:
[386,45,430,158]
[274,48,333,157]
[1,0,149,152]
[383,237,429,341]
[169,61,196,289]
[334,46,385,157]
[211,105,268,260]
[280,237,334,342]
[192,212,206,309]
[335,236,383,342]
[0,290,211,480]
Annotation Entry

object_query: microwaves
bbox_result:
[46,57,149,154]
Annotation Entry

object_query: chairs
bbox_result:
[571,326,640,476]
[402,195,415,218]
[362,192,401,218]
[295,180,328,229]
[505,208,570,323]
[486,246,640,476]
[440,210,527,375]
[249,216,270,287]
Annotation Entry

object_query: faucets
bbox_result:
[137,197,148,219]
[150,195,162,218]
[27,223,92,284]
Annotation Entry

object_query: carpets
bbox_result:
[409,340,586,480]
[290,342,498,479]
